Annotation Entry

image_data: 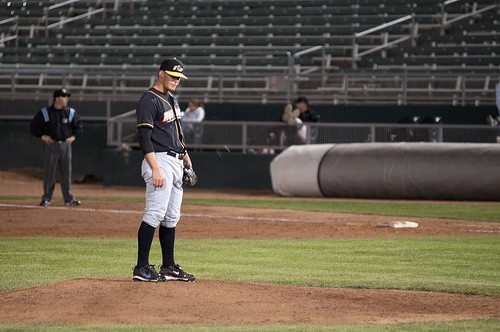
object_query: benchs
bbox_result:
[0,0,500,99]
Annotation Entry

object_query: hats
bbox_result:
[54,89,71,98]
[161,57,189,80]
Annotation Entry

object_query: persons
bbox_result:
[132,57,196,282]
[31,89,82,207]
[181,98,205,134]
[280,96,321,143]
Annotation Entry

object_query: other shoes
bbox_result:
[288,109,300,126]
[65,200,80,206]
[282,104,292,122]
[40,200,50,206]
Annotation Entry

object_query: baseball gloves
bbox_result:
[183,164,198,188]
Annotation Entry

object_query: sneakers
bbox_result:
[159,264,195,281]
[133,264,166,282]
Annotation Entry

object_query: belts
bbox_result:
[167,152,184,160]
